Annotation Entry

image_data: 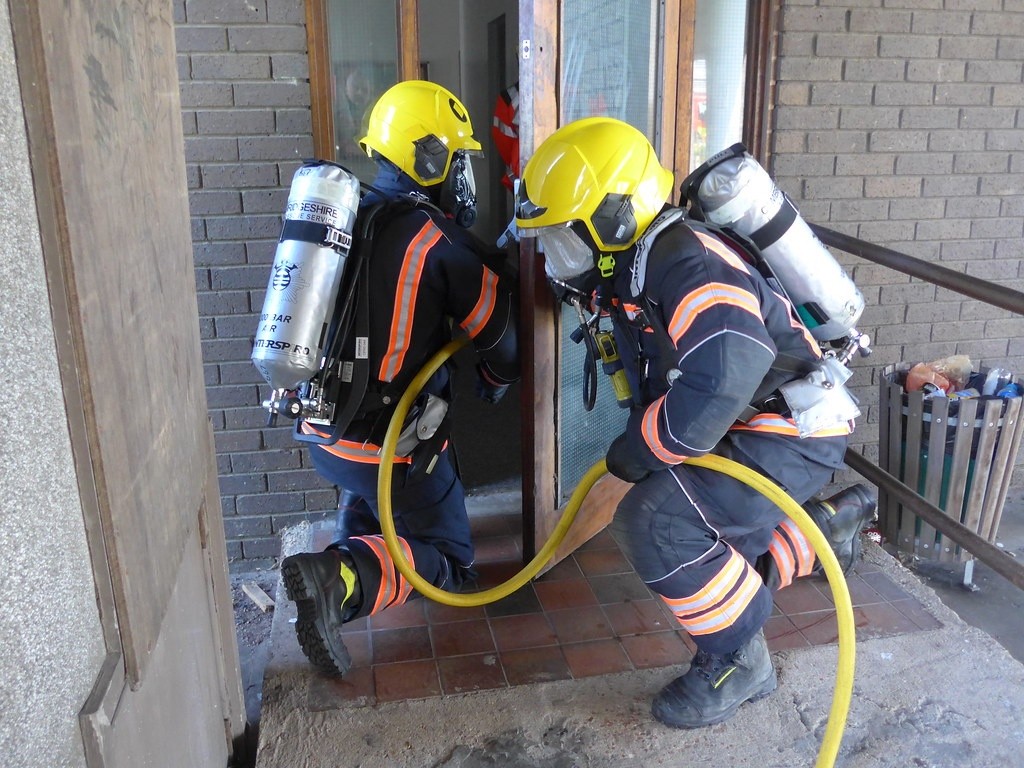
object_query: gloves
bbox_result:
[478,369,508,405]
[607,433,649,482]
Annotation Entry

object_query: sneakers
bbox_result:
[337,491,383,539]
[281,548,356,676]
[814,483,875,581]
[652,628,778,728]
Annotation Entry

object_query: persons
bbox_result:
[487,30,610,200]
[515,116,876,730]
[280,79,513,680]
[336,66,373,149]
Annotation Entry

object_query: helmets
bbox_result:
[513,116,675,251]
[359,79,481,186]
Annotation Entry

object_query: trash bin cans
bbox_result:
[876,360,1024,592]
